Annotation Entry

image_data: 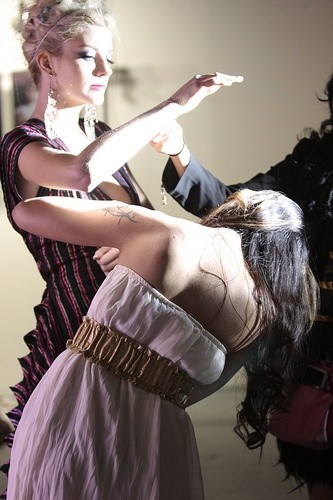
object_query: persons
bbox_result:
[268,384,333,452]
[8,188,319,500]
[150,73,333,500]
[0,0,244,476]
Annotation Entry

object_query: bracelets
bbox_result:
[167,140,185,157]
